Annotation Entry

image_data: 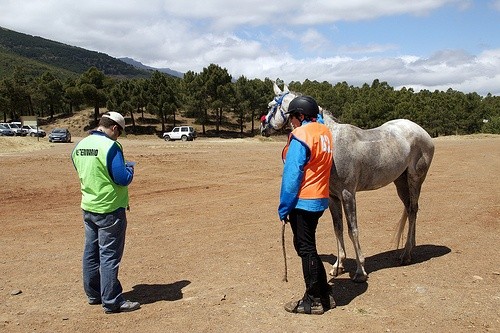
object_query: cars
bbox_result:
[48,128,71,143]
[0,123,21,136]
[21,125,46,138]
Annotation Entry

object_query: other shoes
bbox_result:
[322,292,336,309]
[284,299,324,315]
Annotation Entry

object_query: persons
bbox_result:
[71,112,140,314]
[278,97,336,315]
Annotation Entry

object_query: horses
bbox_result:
[259,82,435,281]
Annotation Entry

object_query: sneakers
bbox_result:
[88,298,102,305]
[105,299,140,314]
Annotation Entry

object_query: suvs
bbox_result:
[162,126,194,141]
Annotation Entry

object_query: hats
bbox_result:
[102,111,125,130]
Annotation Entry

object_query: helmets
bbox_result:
[284,96,319,122]
